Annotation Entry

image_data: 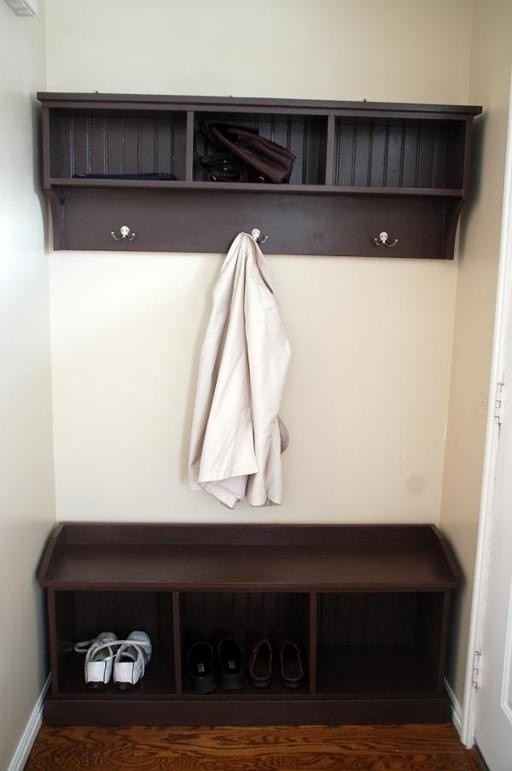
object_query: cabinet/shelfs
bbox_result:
[36,92,482,259]
[38,521,462,726]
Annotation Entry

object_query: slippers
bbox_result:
[274,635,306,689]
[249,637,273,690]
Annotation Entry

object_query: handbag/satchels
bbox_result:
[196,119,296,184]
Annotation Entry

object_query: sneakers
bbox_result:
[75,630,117,693]
[113,629,154,691]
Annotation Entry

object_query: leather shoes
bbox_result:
[215,634,247,691]
[184,632,217,695]
